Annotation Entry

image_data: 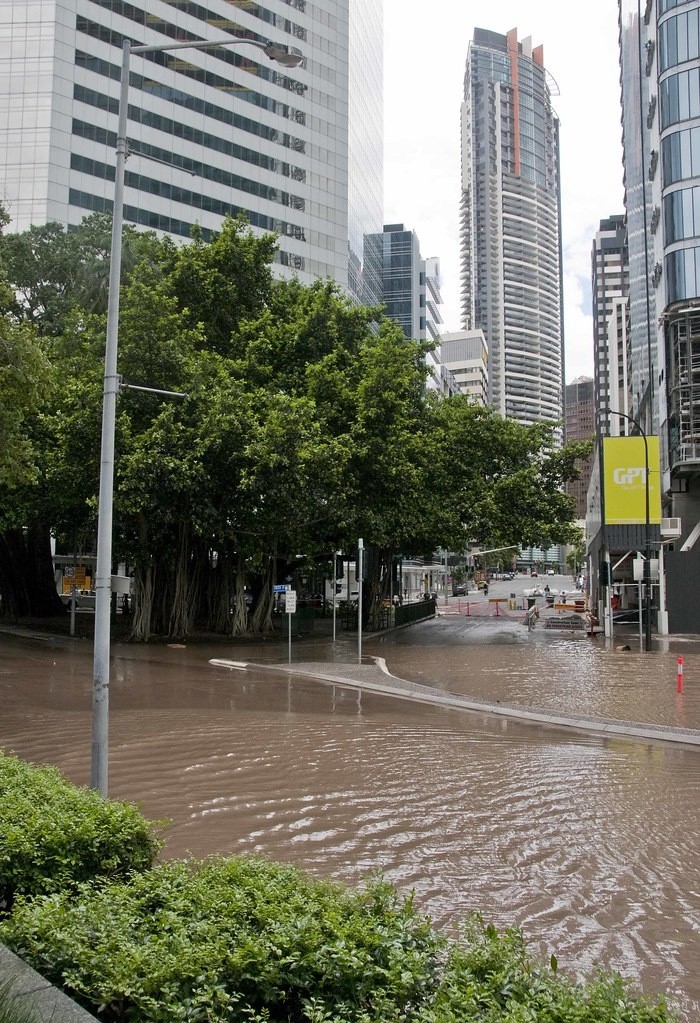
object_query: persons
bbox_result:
[424,591,430,600]
[484,584,488,595]
[544,585,550,596]
[560,591,566,604]
[394,594,403,606]
[431,591,439,609]
[310,592,323,599]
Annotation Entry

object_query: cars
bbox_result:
[531,571,538,577]
[478,581,486,590]
[503,572,514,581]
[547,569,555,576]
[454,585,468,596]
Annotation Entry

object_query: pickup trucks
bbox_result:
[59,588,96,610]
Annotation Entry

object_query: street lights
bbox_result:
[597,406,652,651]
[89,37,308,800]
